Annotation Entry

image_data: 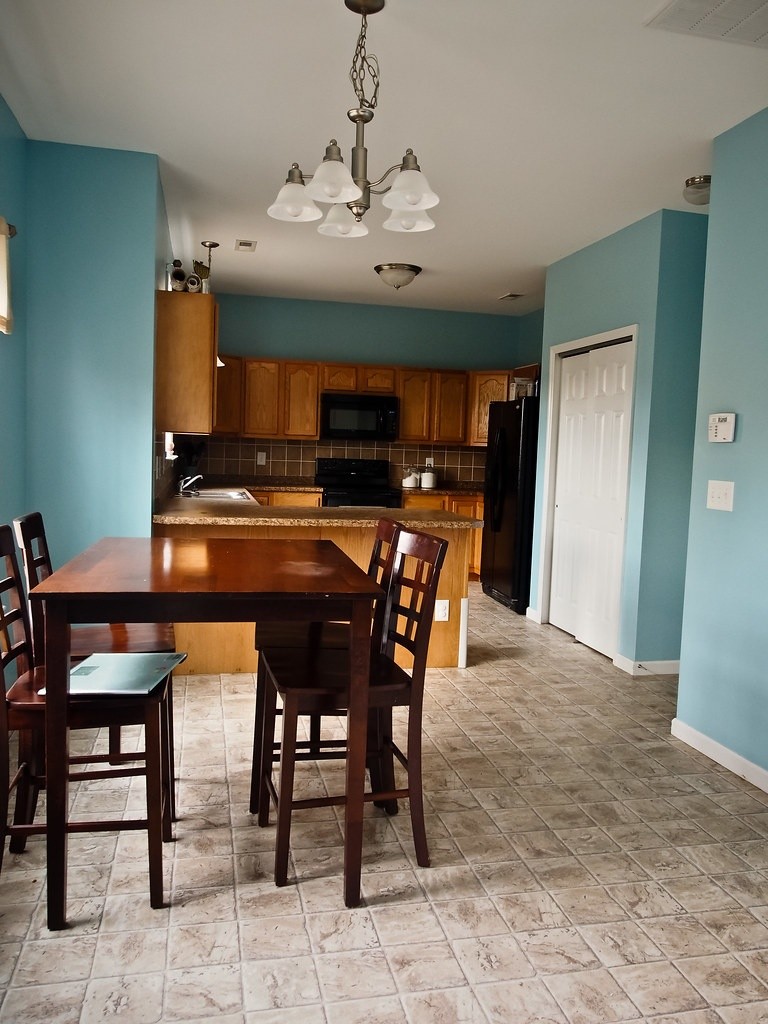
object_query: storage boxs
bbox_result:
[470,367,513,446]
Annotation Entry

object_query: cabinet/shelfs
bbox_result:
[215,352,244,437]
[153,291,218,438]
[406,492,476,575]
[474,496,485,575]
[246,358,321,442]
[321,362,397,397]
[398,366,467,448]
[246,491,322,510]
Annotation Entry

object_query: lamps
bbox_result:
[374,264,423,292]
[266,0,440,240]
[684,174,713,206]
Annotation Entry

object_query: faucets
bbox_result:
[178,474,204,497]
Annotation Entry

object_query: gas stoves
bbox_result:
[313,457,402,508]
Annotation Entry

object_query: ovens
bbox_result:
[319,393,400,442]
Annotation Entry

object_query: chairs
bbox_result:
[0,511,449,815]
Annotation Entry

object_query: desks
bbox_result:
[27,536,386,931]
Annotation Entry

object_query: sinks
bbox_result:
[197,491,229,495]
[195,495,232,499]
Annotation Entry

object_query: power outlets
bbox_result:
[257,451,267,466]
[706,478,735,513]
[425,458,433,470]
[435,600,449,622]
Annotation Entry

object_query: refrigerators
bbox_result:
[480,394,540,614]
